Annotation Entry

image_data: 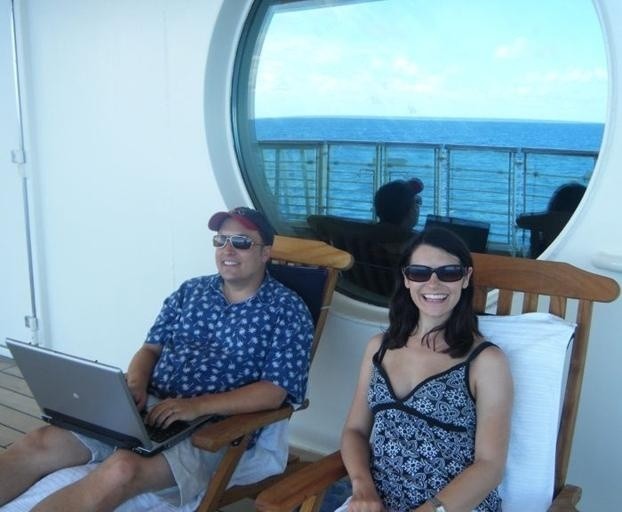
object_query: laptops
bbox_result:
[5,338,216,458]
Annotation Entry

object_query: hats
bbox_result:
[374,178,424,225]
[207,205,275,245]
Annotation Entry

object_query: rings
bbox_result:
[169,405,177,413]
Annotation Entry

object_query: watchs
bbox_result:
[428,495,448,512]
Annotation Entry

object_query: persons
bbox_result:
[347,174,426,249]
[515,178,588,258]
[0,205,316,511]
[316,227,518,512]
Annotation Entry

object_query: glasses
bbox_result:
[212,234,265,250]
[401,263,466,282]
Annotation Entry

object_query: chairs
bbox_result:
[0,236,355,511]
[254,252,622,511]
[307,215,568,299]
